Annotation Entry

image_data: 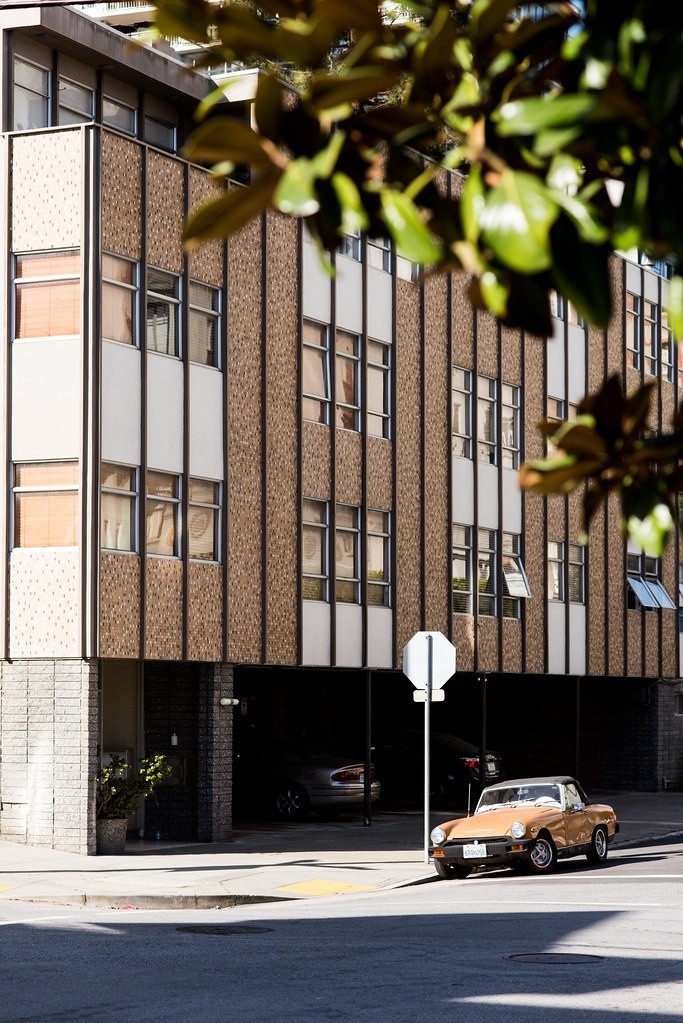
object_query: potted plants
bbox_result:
[93,752,174,856]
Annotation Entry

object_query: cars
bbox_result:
[260,742,381,823]
[372,729,513,811]
[427,774,622,880]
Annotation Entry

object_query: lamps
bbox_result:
[220,697,239,706]
